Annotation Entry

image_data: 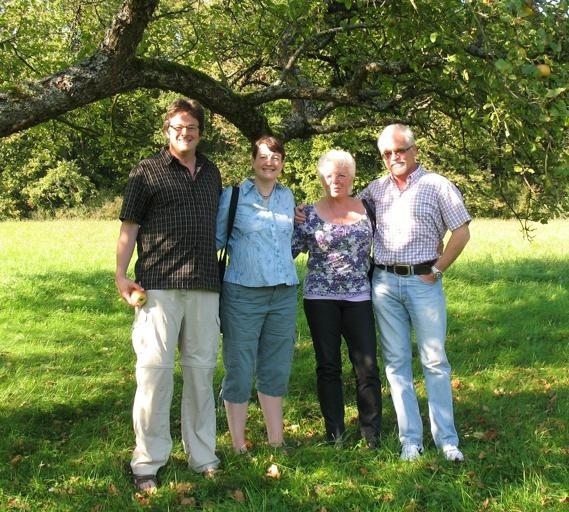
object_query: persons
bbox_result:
[114,97,222,493]
[214,135,301,455]
[291,149,382,448]
[295,122,472,463]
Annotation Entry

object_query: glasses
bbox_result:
[381,145,412,160]
[169,124,199,132]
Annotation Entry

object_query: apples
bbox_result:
[536,64,550,78]
[130,290,146,306]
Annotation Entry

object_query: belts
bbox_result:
[375,259,437,277]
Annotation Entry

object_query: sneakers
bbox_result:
[400,443,424,461]
[443,444,464,461]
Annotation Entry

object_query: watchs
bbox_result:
[430,265,444,280]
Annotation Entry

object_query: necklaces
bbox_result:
[259,184,275,198]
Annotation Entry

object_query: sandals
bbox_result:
[132,475,158,488]
